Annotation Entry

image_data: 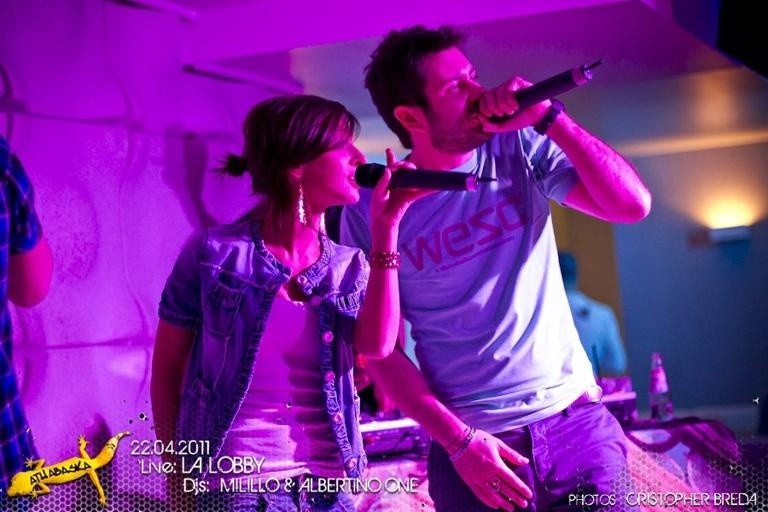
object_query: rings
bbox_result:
[487,477,501,494]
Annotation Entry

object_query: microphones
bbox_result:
[355,163,497,193]
[475,57,604,124]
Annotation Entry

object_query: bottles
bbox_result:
[649,351,674,422]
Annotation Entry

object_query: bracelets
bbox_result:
[444,423,477,462]
[367,249,402,271]
[532,97,567,137]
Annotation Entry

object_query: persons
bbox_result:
[553,249,630,383]
[0,59,56,510]
[336,20,657,512]
[147,93,439,511]
